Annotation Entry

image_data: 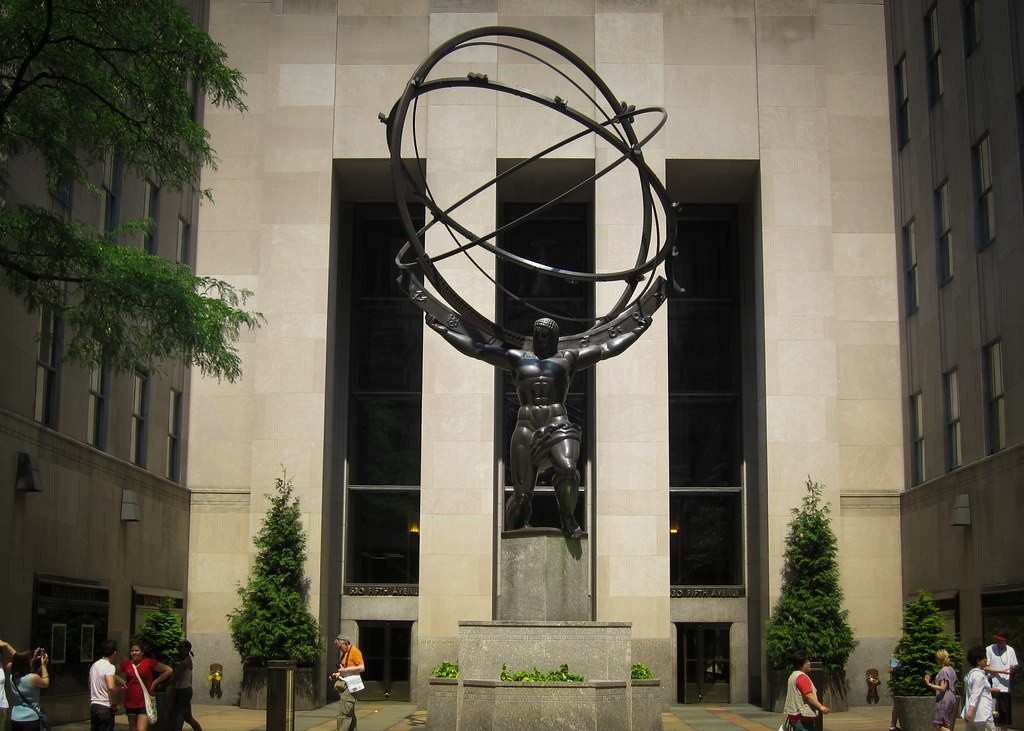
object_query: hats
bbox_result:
[992,630,1009,640]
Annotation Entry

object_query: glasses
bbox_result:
[336,637,347,642]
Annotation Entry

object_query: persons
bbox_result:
[0,638,203,731]
[887,630,1019,731]
[782,653,829,731]
[329,633,365,731]
[426,313,654,539]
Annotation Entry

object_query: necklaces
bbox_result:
[996,645,1008,665]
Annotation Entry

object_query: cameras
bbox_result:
[39,648,45,658]
[336,664,344,670]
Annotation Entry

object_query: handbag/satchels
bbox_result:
[777,717,792,731]
[39,712,51,731]
[144,696,158,725]
[339,674,365,693]
[334,680,347,692]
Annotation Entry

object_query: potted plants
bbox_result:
[764,475,848,712]
[129,595,186,731]
[886,590,962,731]
[225,463,325,712]
